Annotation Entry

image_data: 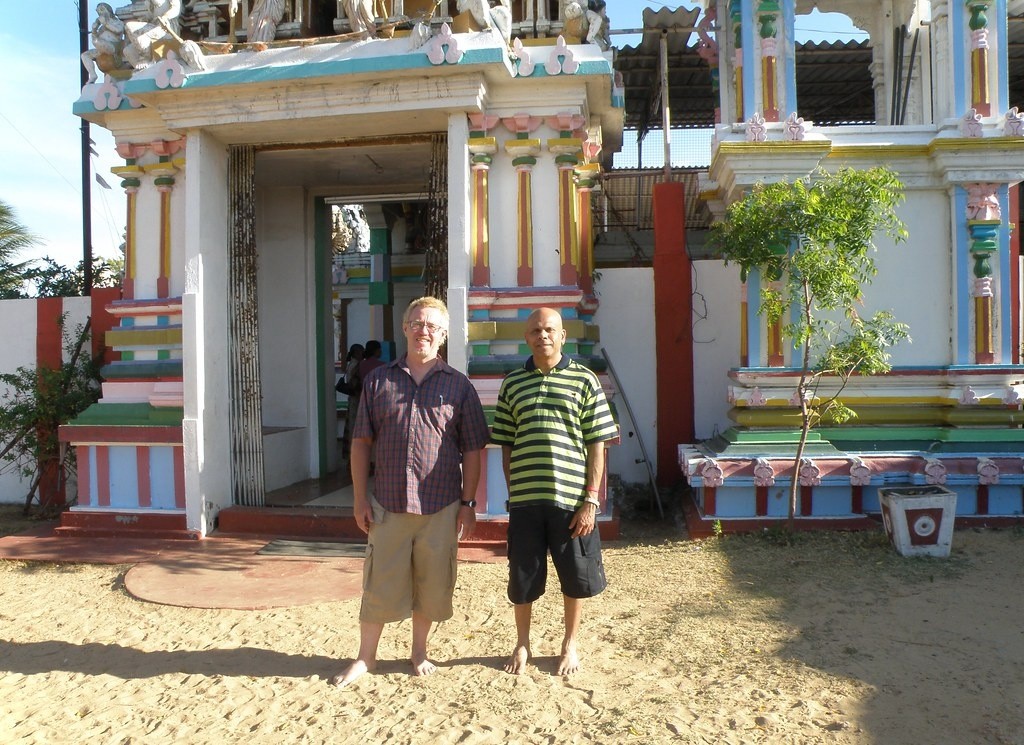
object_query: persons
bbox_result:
[331,295,490,685]
[341,340,386,480]
[489,305,620,676]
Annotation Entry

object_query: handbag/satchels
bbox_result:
[335,361,354,397]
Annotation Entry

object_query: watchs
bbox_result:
[460,499,480,509]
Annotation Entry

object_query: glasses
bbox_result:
[406,320,445,334]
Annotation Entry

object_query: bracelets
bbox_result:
[582,495,603,507]
